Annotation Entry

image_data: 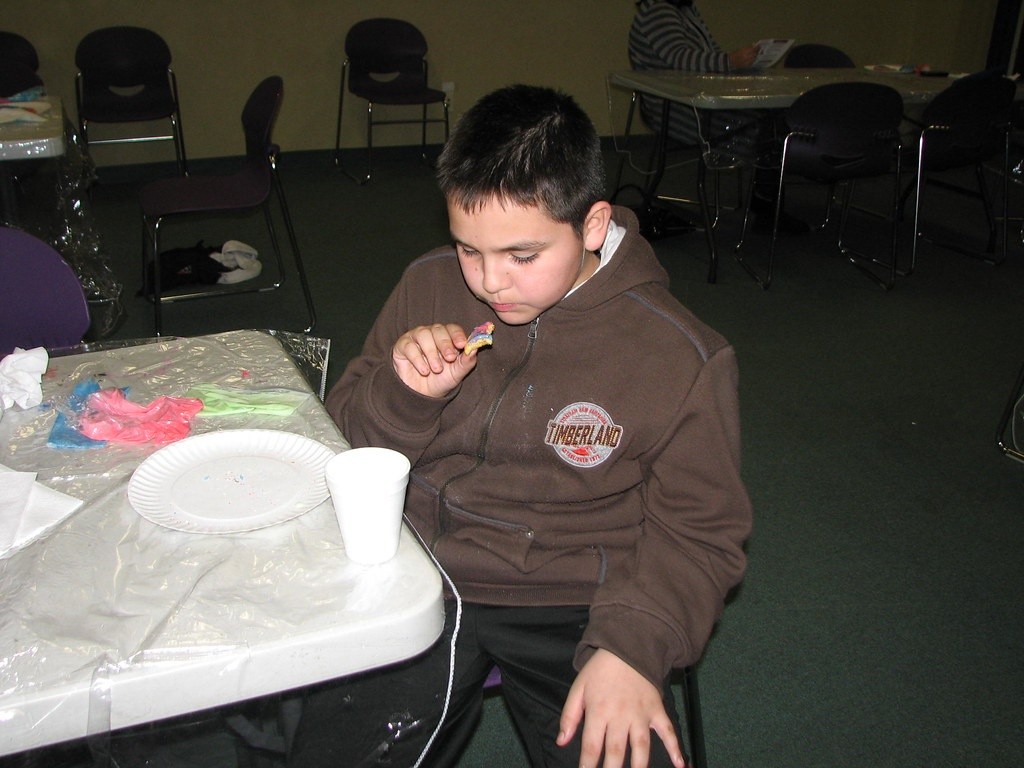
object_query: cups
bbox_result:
[325,447,411,563]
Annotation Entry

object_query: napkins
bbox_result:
[0,347,48,410]
[0,463,83,557]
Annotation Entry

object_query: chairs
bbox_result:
[143,75,316,335]
[0,31,45,100]
[611,45,1024,293]
[74,25,187,178]
[335,17,450,188]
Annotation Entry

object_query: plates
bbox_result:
[128,430,336,533]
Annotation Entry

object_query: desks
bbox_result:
[608,64,1024,284]
[0,96,66,160]
[0,330,444,757]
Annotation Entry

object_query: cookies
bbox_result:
[464,322,494,355]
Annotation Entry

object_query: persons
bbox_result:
[629,1,807,237]
[285,81,753,763]
